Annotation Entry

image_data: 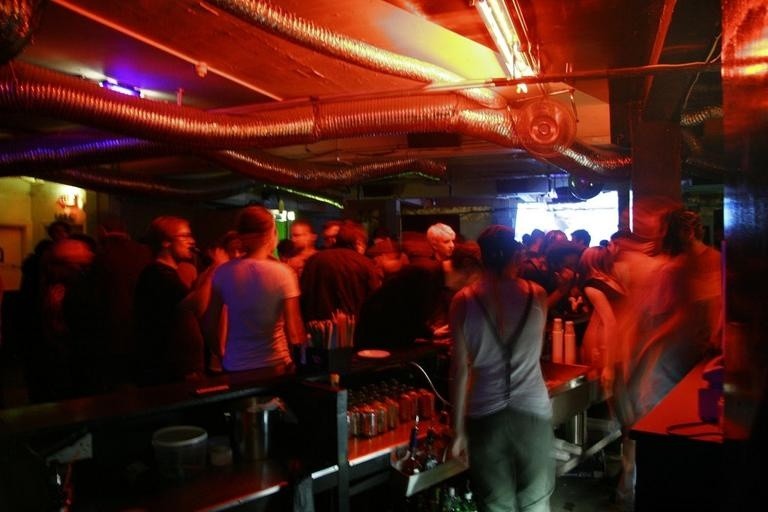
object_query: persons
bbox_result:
[522,195,724,509]
[452,225,555,512]
[17,199,477,383]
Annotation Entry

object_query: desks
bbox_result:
[628,353,755,512]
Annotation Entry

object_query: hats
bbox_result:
[477,225,523,252]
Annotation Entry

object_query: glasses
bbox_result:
[173,233,193,241]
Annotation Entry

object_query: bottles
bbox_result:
[552,318,564,364]
[564,321,577,365]
[423,433,438,470]
[463,493,478,512]
[430,411,452,458]
[442,487,462,511]
[437,432,453,466]
[402,427,423,473]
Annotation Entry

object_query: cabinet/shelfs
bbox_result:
[301,319,621,512]
[4,381,339,512]
